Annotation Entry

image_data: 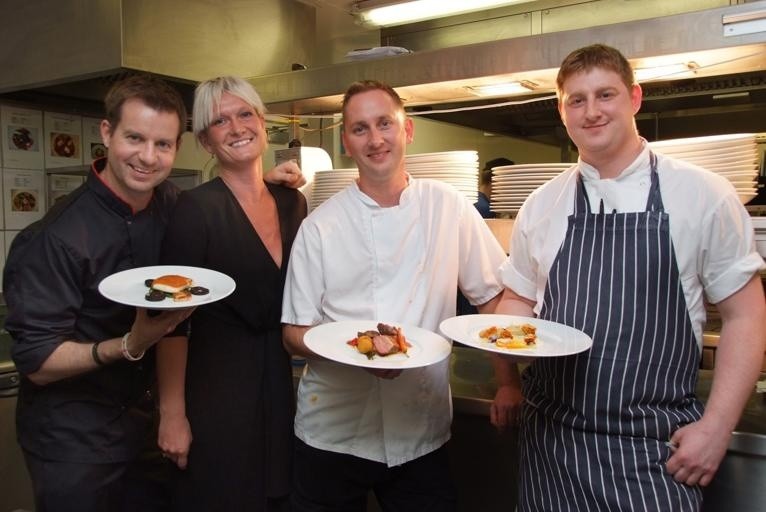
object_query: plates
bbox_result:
[488,163,578,217]
[11,189,39,212]
[401,150,480,205]
[98,265,237,311]
[91,144,107,159]
[439,314,593,358]
[310,168,360,213]
[648,132,760,204]
[10,127,36,151]
[51,192,68,207]
[51,133,77,159]
[303,320,452,370]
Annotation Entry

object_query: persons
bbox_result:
[277,78,526,510]
[474,157,516,218]
[0,69,307,512]
[151,74,308,512]
[492,42,766,511]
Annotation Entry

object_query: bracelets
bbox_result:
[92,342,110,367]
[121,332,146,362]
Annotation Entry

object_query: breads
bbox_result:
[151,274,192,294]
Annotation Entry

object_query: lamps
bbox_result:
[351,0,544,31]
